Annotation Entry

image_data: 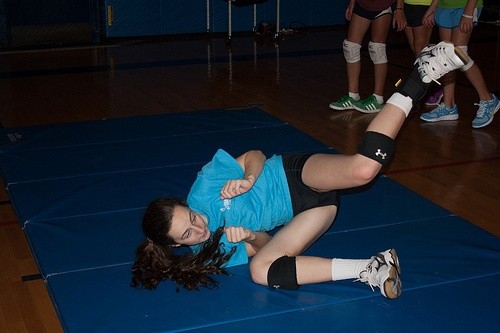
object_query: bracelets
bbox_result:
[396,8,404,10]
[463,14,473,18]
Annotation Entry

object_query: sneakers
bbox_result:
[472,94,500,127]
[351,95,385,113]
[413,40,469,85]
[352,249,401,299]
[420,102,459,121]
[425,89,444,105]
[329,95,362,110]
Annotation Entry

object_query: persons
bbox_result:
[330,0,407,113]
[422,0,500,128]
[401,0,443,105]
[131,41,469,298]
